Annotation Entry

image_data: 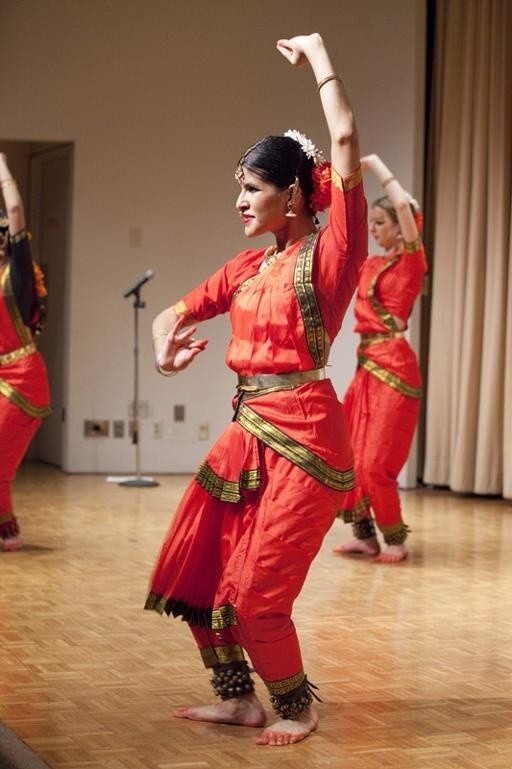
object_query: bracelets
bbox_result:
[382,177,396,188]
[0,177,18,187]
[154,361,178,378]
[318,75,346,91]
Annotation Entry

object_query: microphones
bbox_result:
[123,270,154,298]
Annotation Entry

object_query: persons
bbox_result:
[0,153,51,550]
[335,153,428,562]
[149,33,367,747]
